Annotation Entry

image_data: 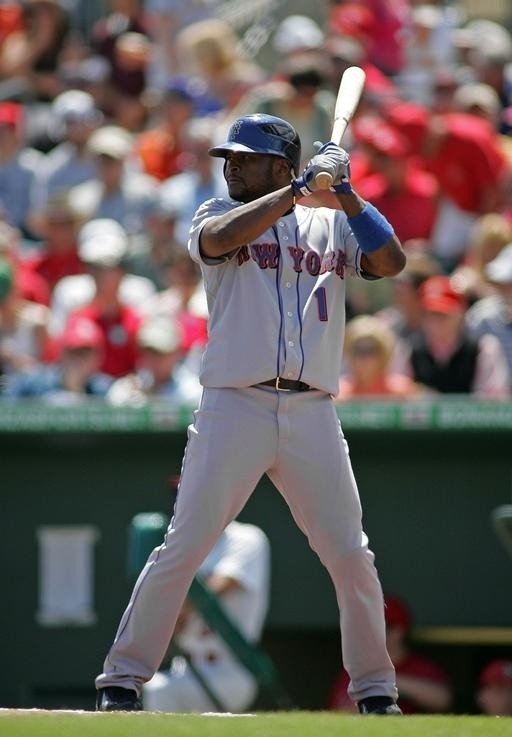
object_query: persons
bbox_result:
[475,657,510,716]
[139,473,275,713]
[329,592,453,715]
[2,0,510,409]
[95,111,403,718]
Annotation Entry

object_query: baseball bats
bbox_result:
[316,67,366,190]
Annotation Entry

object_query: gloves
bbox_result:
[291,141,353,196]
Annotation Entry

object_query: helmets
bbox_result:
[208,114,301,162]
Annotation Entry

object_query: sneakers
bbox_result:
[357,696,402,715]
[96,686,143,710]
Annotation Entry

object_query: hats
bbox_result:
[275,15,321,49]
[381,594,413,632]
[478,659,512,688]
[5,4,204,356]
[449,19,511,115]
[346,241,511,364]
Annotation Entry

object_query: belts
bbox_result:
[260,377,308,392]
